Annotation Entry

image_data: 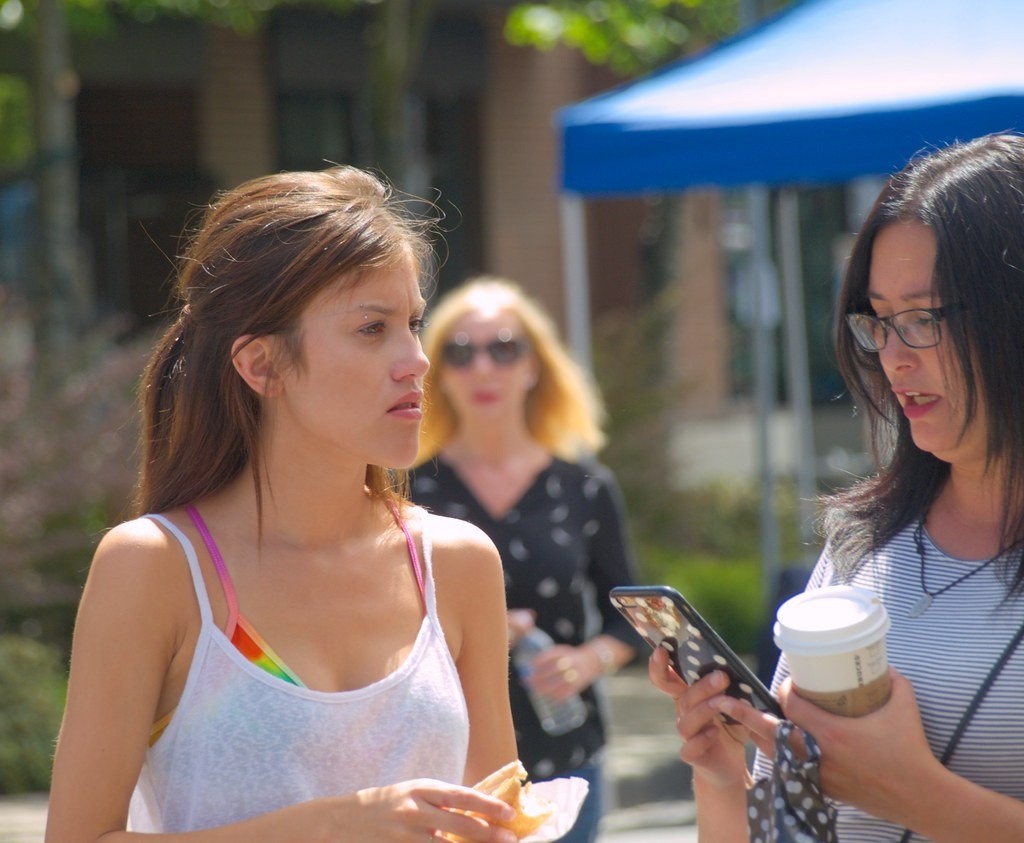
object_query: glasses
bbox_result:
[439,341,531,365]
[844,302,970,352]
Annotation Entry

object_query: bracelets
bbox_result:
[593,639,614,675]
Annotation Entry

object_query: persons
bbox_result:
[44,162,523,843]
[398,278,648,843]
[647,132,1024,842]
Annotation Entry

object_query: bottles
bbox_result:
[509,610,588,736]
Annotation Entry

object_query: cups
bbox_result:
[773,585,892,718]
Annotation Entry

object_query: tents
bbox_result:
[553,0,1024,588]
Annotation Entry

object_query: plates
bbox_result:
[434,776,590,843]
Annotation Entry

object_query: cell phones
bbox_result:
[608,585,787,724]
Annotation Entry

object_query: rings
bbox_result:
[559,657,578,685]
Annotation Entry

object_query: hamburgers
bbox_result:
[439,760,556,843]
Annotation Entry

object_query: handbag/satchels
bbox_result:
[748,721,838,843]
